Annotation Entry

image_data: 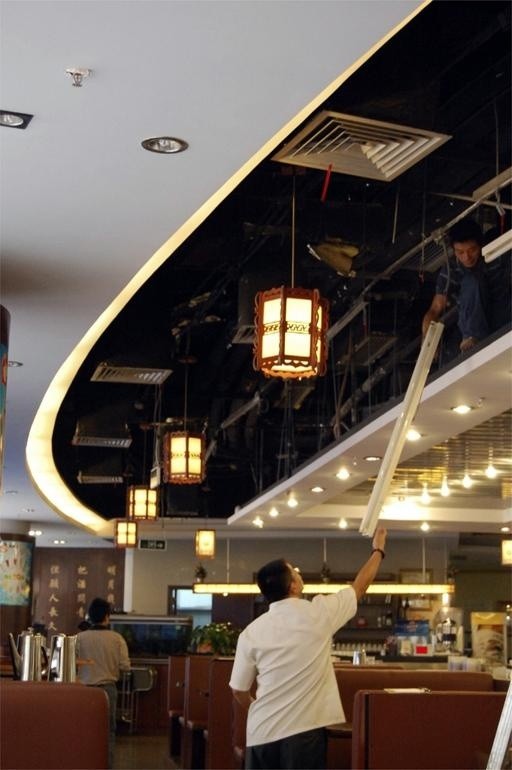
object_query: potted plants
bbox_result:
[320,561,332,583]
[193,561,208,583]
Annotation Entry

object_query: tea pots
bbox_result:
[7,627,79,684]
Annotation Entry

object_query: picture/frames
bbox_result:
[398,568,433,610]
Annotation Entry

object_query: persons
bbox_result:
[421,224,512,363]
[76,598,130,770]
[229,526,387,770]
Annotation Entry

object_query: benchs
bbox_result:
[351,688,512,769]
[168,655,494,769]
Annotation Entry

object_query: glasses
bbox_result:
[293,566,300,578]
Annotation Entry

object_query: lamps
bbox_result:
[251,189,330,383]
[114,373,218,558]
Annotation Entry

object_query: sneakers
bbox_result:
[459,336,478,353]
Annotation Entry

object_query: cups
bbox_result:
[353,649,366,665]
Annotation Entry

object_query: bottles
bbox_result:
[378,597,409,627]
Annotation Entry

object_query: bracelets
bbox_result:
[371,549,385,559]
